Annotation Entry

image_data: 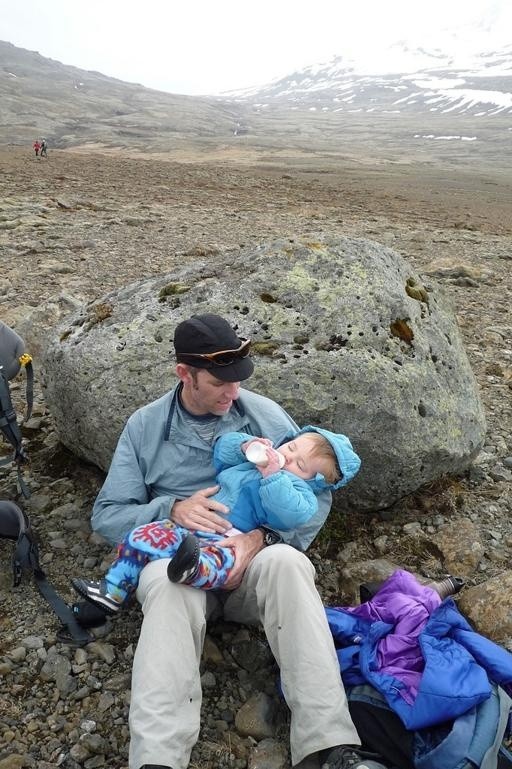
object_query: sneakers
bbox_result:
[166,533,202,585]
[319,747,388,768]
[69,577,124,614]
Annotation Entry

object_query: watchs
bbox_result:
[260,525,276,545]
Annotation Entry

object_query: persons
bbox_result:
[72,424,361,614]
[90,314,385,769]
[33,138,48,157]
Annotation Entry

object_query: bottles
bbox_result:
[422,576,464,597]
[244,442,285,470]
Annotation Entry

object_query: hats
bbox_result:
[174,313,255,384]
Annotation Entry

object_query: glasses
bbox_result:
[177,336,251,368]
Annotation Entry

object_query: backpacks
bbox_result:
[346,680,511,769]
